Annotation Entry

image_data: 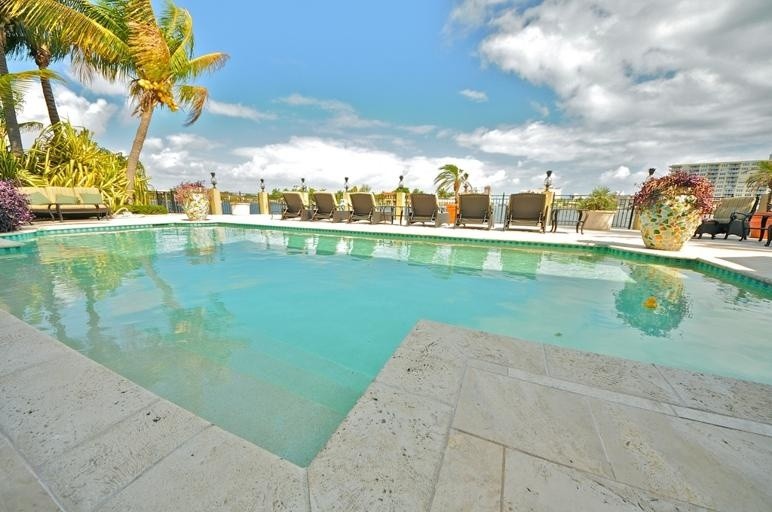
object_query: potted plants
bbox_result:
[577,186,619,230]
[229,195,251,215]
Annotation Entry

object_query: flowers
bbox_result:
[631,168,716,213]
[186,247,218,256]
[173,180,205,205]
[616,282,690,337]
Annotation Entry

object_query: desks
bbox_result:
[550,208,590,234]
[736,212,772,246]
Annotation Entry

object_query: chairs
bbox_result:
[407,193,450,228]
[503,193,548,233]
[347,193,392,224]
[693,196,759,239]
[455,194,494,230]
[311,193,353,222]
[283,232,543,282]
[280,193,316,220]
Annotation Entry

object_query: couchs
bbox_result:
[17,186,108,223]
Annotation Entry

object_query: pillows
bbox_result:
[55,194,79,205]
[29,192,50,204]
[79,193,103,205]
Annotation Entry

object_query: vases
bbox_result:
[188,227,214,249]
[626,265,685,303]
[181,190,210,221]
[639,194,702,251]
